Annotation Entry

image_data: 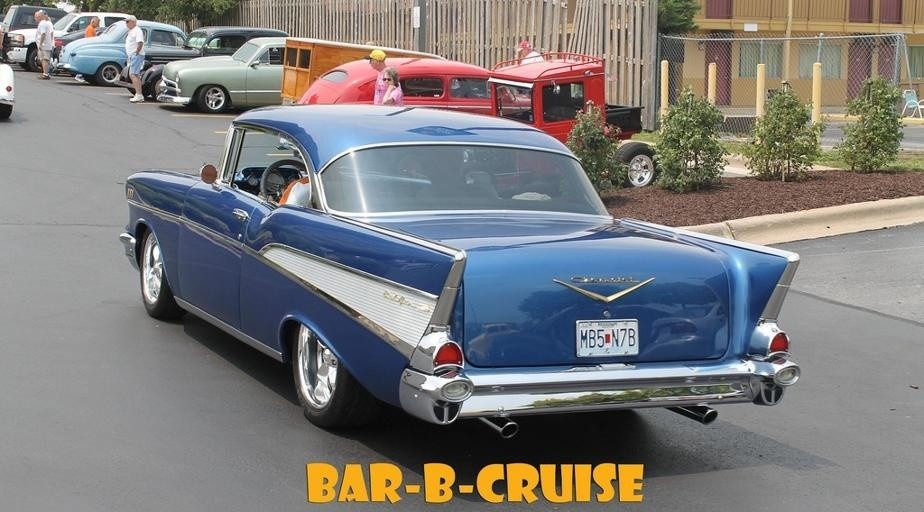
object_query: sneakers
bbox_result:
[129,94,144,103]
[36,73,49,80]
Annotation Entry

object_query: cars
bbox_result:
[155,36,320,115]
[118,103,801,439]
[114,25,290,101]
[0,3,189,120]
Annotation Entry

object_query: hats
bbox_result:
[361,50,386,61]
[122,15,136,22]
[516,41,532,52]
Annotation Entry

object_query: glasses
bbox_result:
[382,79,391,82]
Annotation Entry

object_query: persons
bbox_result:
[121,15,146,104]
[382,69,402,107]
[33,10,54,81]
[365,49,390,103]
[39,10,55,79]
[73,16,101,84]
[516,41,547,66]
[258,175,313,209]
